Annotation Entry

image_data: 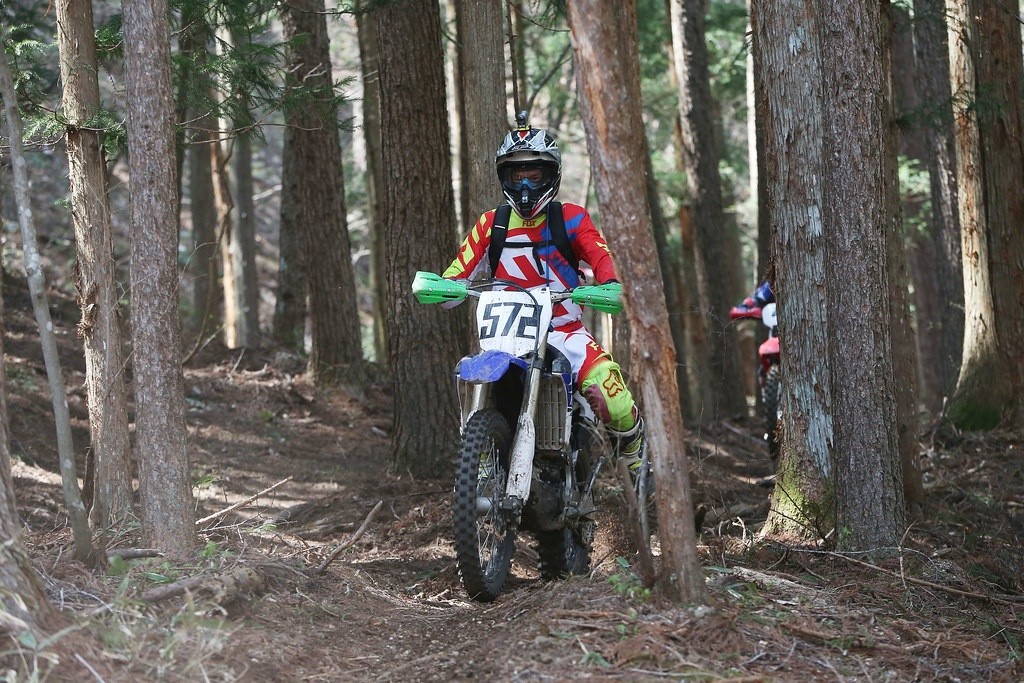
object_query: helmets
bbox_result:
[495,110,562,220]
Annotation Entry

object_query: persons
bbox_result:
[737,281,776,311]
[441,125,646,485]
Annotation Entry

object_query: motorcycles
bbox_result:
[411,269,624,603]
[727,300,782,461]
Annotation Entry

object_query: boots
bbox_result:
[604,405,657,506]
